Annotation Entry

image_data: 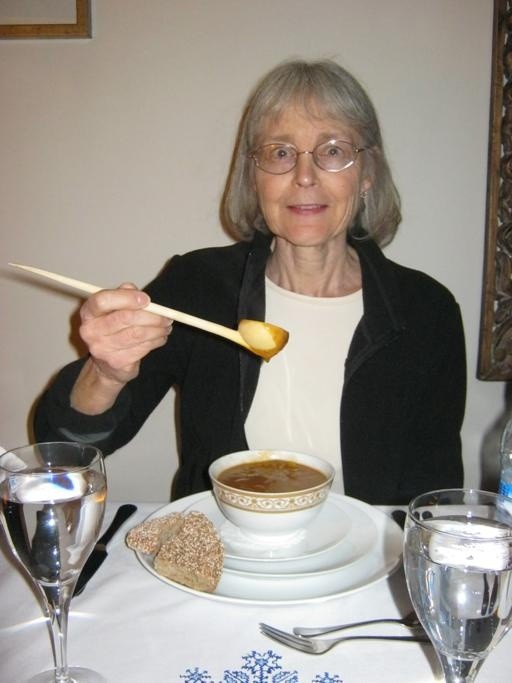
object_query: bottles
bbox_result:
[494,418,511,530]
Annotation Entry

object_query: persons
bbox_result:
[32,54,467,508]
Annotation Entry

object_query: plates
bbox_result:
[134,490,403,609]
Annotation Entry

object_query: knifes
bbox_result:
[72,503,137,601]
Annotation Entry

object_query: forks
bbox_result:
[259,622,429,655]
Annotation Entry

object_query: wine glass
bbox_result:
[0,441,106,683]
[404,488,511,682]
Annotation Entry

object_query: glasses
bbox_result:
[248,140,367,175]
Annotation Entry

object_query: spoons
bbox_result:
[6,258,289,360]
[293,610,423,637]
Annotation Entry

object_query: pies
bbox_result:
[154,509,222,591]
[127,513,183,555]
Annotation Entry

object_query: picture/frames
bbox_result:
[1,0,95,40]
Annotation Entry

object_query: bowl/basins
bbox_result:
[208,448,335,529]
